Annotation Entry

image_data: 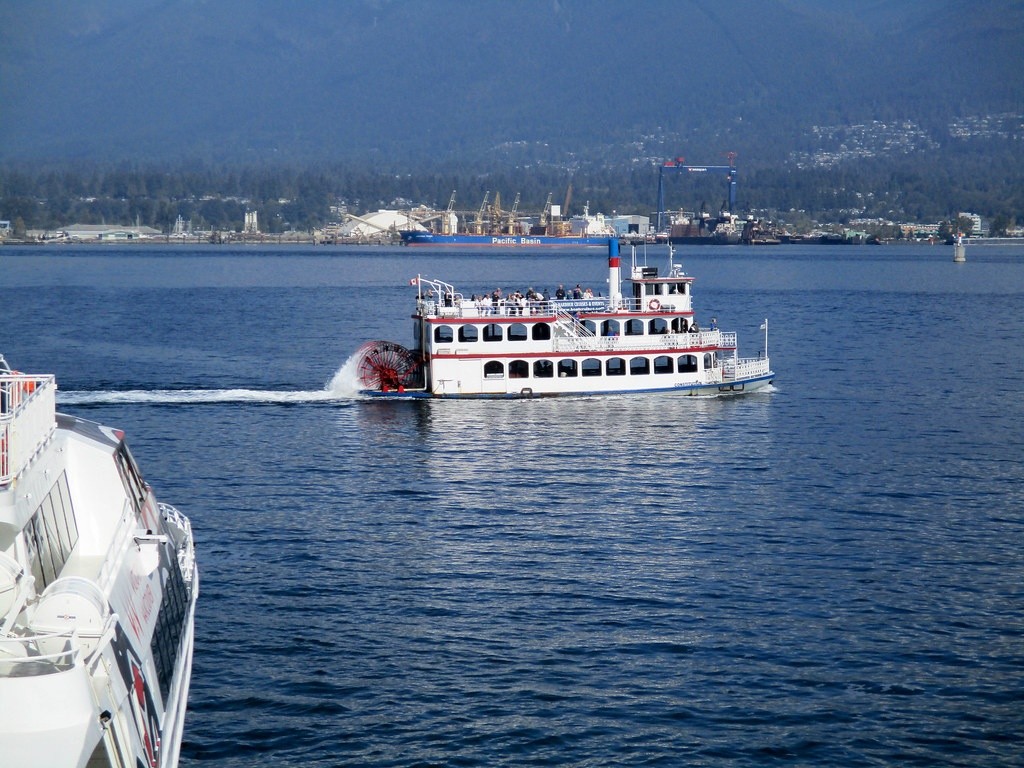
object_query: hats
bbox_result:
[577,284,580,288]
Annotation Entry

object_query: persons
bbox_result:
[661,320,687,334]
[542,290,550,301]
[583,288,593,298]
[556,285,565,300]
[543,361,549,368]
[608,327,619,336]
[526,287,539,313]
[567,290,573,299]
[688,321,699,333]
[415,290,433,299]
[710,319,717,330]
[507,290,524,314]
[471,287,502,314]
[574,284,583,299]
[444,289,451,307]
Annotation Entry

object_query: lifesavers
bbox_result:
[649,299,661,310]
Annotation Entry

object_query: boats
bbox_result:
[0,355,206,768]
[346,225,778,399]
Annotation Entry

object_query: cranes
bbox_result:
[440,188,553,237]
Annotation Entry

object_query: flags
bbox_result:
[409,277,419,285]
